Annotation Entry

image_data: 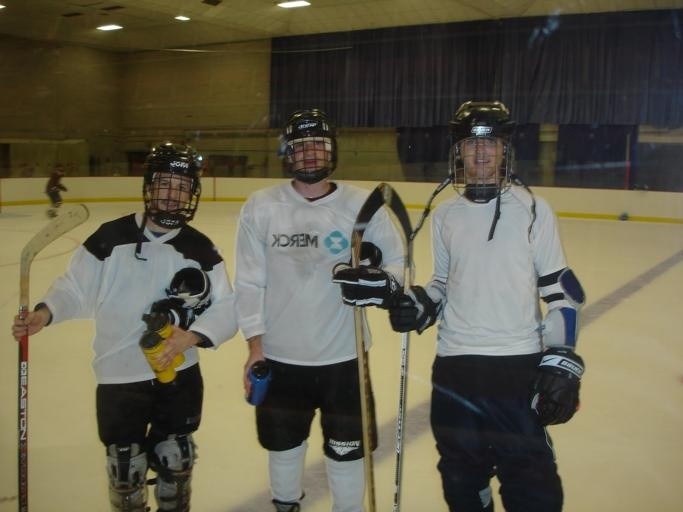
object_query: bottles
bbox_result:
[243,360,272,407]
[138,330,178,385]
[141,311,186,369]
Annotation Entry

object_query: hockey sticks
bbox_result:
[381,184,415,512]
[17,204,88,511]
[351,183,387,511]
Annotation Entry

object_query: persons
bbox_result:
[386,98,577,510]
[237,110,405,508]
[11,139,237,512]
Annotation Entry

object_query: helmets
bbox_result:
[143,141,202,191]
[282,109,337,159]
[450,99,514,160]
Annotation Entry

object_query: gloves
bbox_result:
[332,242,437,335]
[527,347,585,425]
[142,267,212,331]
